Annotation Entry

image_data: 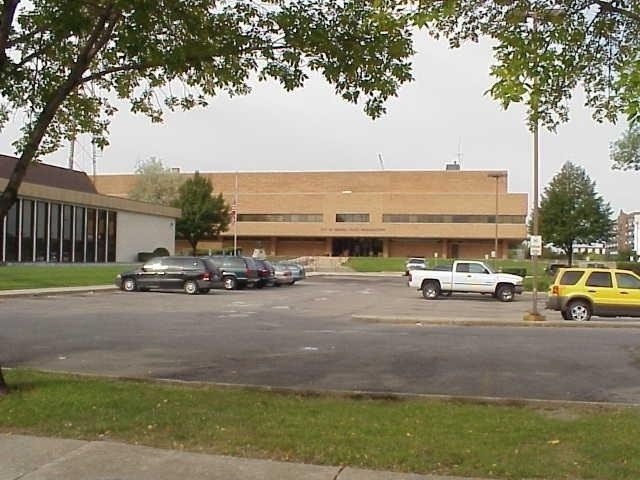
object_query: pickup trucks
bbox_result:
[407,260,527,301]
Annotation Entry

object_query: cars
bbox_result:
[114,255,224,295]
[545,267,640,321]
[209,254,307,295]
[405,257,426,276]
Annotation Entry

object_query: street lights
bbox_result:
[504,5,567,319]
